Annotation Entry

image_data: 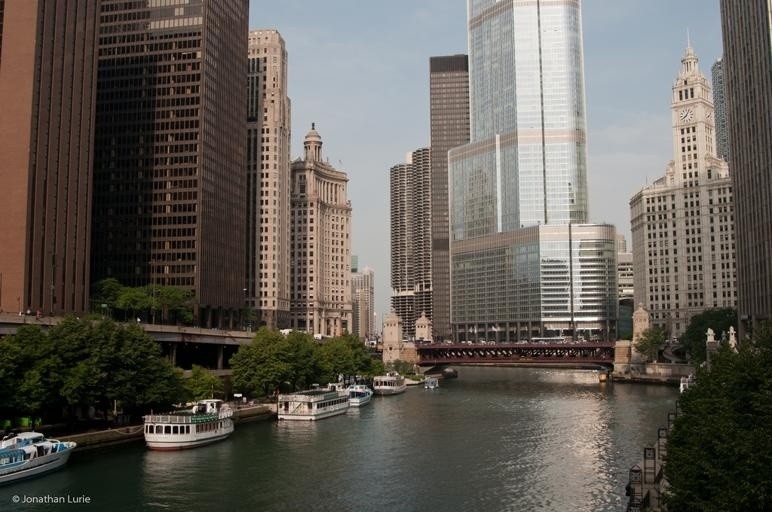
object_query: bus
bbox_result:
[528,337,565,345]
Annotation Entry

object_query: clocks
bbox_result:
[679,108,693,123]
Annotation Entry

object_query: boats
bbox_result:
[0,430,77,482]
[373,371,406,395]
[345,382,374,407]
[142,399,234,451]
